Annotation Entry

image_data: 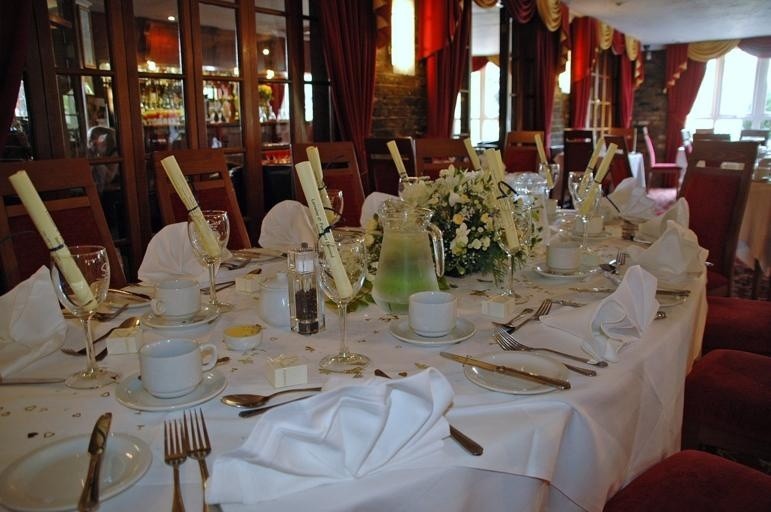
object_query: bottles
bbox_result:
[290,242,326,334]
[136,77,185,124]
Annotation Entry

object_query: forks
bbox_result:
[505,299,553,336]
[182,409,223,510]
[615,250,628,276]
[163,418,188,512]
[496,325,608,378]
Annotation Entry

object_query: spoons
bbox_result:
[62,280,152,362]
[202,268,261,293]
[599,258,616,272]
[220,385,322,409]
[492,308,533,326]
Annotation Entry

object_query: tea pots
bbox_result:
[374,197,445,316]
[254,271,291,329]
[511,172,550,253]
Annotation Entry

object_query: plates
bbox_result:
[465,350,571,392]
[117,369,226,410]
[581,235,611,241]
[634,236,652,244]
[534,264,589,278]
[1,432,152,511]
[389,317,476,347]
[145,300,222,328]
[238,248,281,261]
[657,296,687,308]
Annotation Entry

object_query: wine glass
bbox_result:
[495,205,530,304]
[539,163,604,255]
[47,246,118,389]
[317,230,369,373]
[265,143,292,164]
[185,209,232,313]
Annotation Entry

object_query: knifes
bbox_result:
[2,376,64,387]
[557,299,665,323]
[79,410,113,512]
[228,249,286,260]
[440,350,570,391]
[568,287,690,294]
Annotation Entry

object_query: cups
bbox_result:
[399,176,434,204]
[410,291,458,337]
[318,189,343,227]
[140,338,217,399]
[546,243,584,273]
[151,279,201,319]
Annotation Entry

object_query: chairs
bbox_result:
[673,137,760,296]
[411,136,474,179]
[503,127,547,173]
[291,140,368,228]
[682,345,771,440]
[563,117,771,288]
[600,448,771,511]
[3,151,125,311]
[153,144,254,259]
[703,286,771,354]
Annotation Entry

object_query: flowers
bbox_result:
[399,168,521,283]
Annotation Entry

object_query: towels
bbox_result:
[359,189,403,231]
[1,263,72,385]
[641,195,692,243]
[135,220,224,287]
[204,364,458,506]
[257,198,337,259]
[537,264,663,366]
[631,222,718,289]
[600,176,655,220]
